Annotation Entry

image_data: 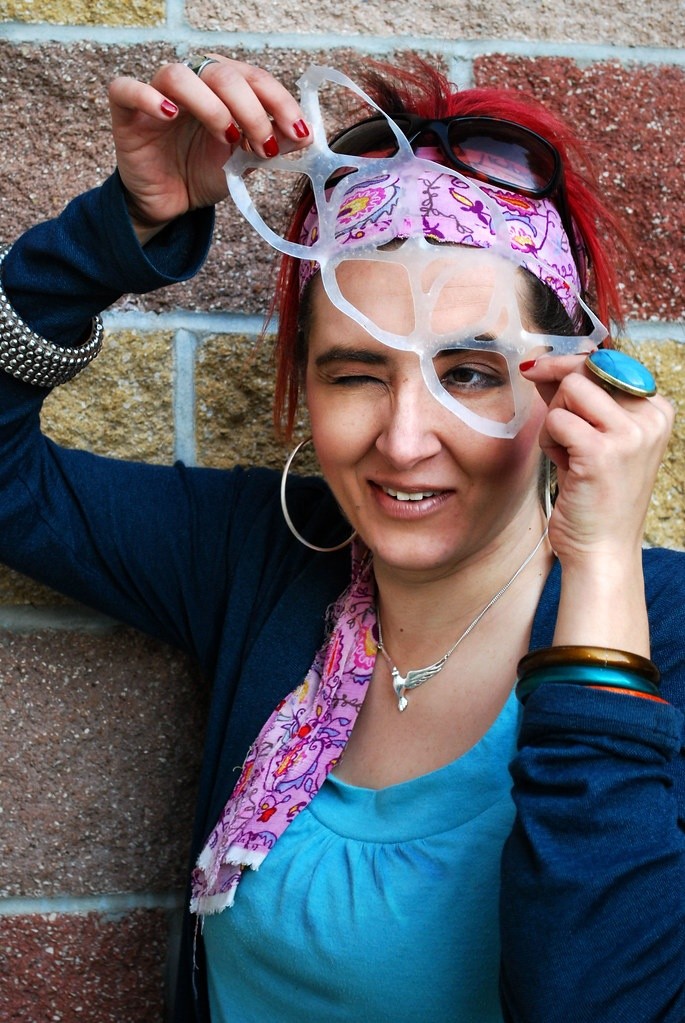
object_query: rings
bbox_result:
[182,54,221,78]
[585,349,657,398]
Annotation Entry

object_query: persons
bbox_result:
[1,49,685,1023]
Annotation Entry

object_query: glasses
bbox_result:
[298,112,587,305]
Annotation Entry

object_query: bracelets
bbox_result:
[0,243,105,388]
[514,645,661,706]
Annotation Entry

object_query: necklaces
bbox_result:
[376,524,548,712]
